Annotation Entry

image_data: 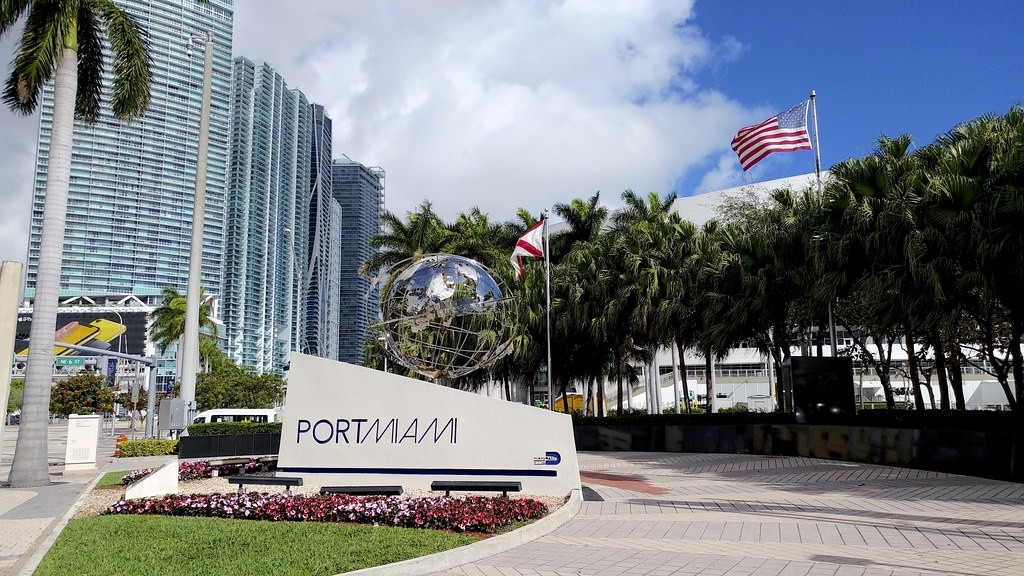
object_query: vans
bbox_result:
[179,409,274,440]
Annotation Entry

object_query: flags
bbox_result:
[509,218,546,282]
[731,99,812,172]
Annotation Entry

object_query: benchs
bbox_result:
[320,486,403,498]
[228,476,303,494]
[431,481,522,497]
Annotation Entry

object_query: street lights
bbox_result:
[158,28,217,448]
